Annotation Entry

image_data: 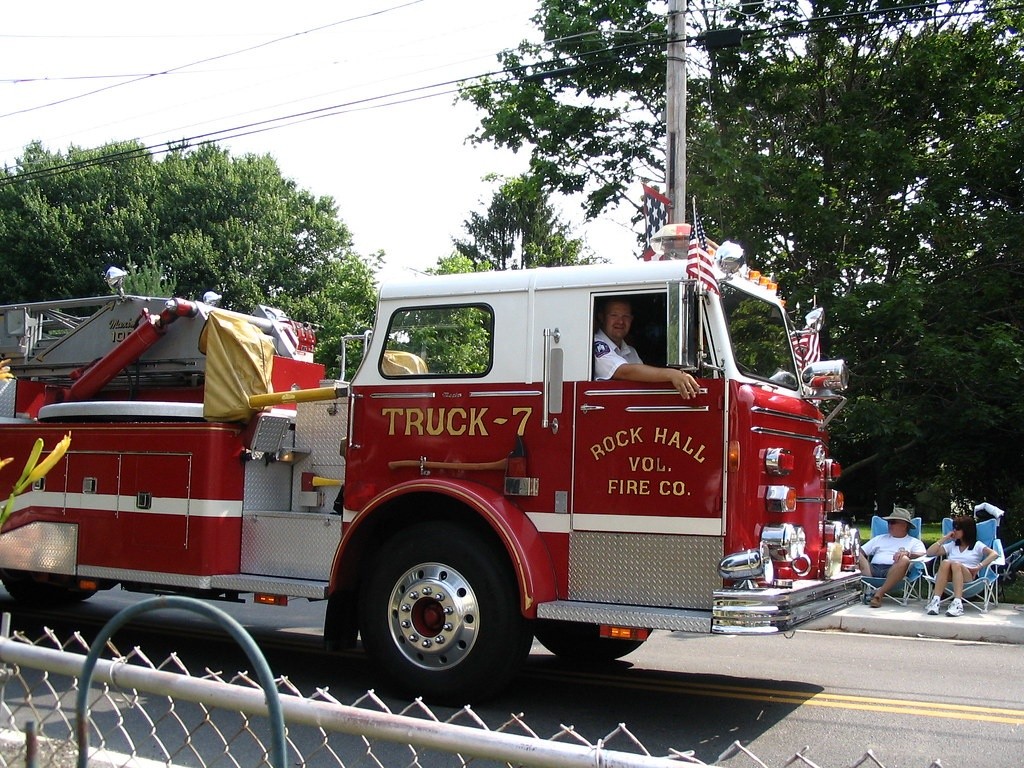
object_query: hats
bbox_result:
[882,508,917,529]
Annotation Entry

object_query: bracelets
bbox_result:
[978,563,984,570]
[936,542,941,546]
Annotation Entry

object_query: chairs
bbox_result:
[921,518,1006,614]
[860,515,923,607]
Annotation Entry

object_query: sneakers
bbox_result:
[945,604,964,616]
[927,601,940,615]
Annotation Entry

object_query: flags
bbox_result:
[641,183,671,262]
[686,205,721,295]
[789,321,821,373]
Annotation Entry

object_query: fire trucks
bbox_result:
[0,241,864,705]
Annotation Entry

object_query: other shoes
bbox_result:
[870,595,881,607]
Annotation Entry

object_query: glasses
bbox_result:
[888,521,905,525]
[955,526,961,531]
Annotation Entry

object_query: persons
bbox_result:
[592,296,700,401]
[857,508,928,608]
[926,516,998,617]
